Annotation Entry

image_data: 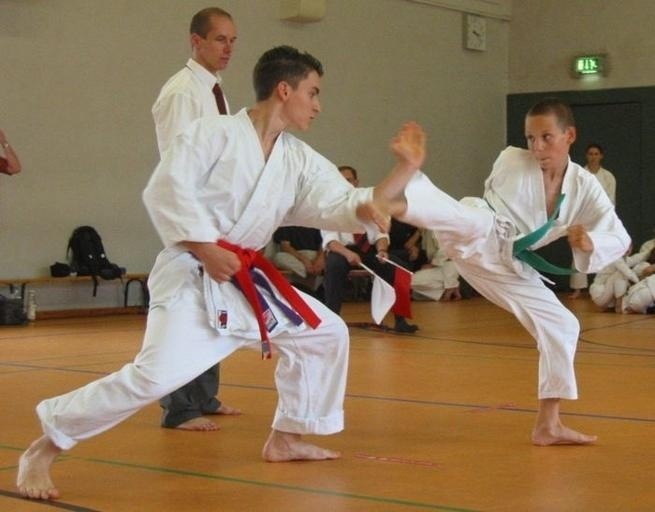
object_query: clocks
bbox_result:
[465,14,487,52]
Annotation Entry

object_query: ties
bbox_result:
[213,84,227,115]
[354,233,370,253]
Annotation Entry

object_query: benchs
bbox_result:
[0,272,149,318]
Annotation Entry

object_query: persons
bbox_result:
[272,226,324,305]
[318,165,417,333]
[0,128,21,176]
[17,46,425,499]
[357,99,635,447]
[374,216,474,302]
[569,144,655,316]
[151,7,242,432]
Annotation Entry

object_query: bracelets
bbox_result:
[2,141,10,149]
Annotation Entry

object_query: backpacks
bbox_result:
[66,226,108,298]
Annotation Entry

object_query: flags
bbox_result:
[371,276,397,325]
[393,267,413,319]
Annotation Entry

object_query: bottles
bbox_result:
[26,291,36,321]
